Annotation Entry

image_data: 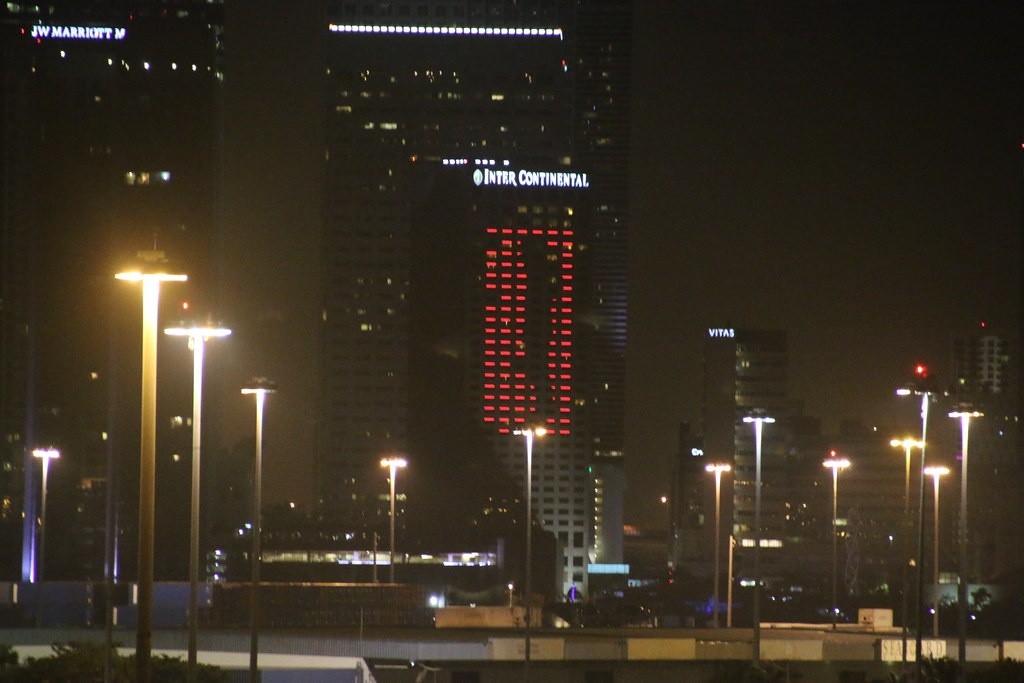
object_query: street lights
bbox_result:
[947,409,986,664]
[240,387,274,683]
[890,435,928,663]
[33,447,61,581]
[513,426,547,660]
[163,325,232,683]
[922,465,952,639]
[743,414,777,664]
[115,267,188,683]
[706,463,732,630]
[822,458,851,631]
[895,381,947,683]
[380,456,407,584]
